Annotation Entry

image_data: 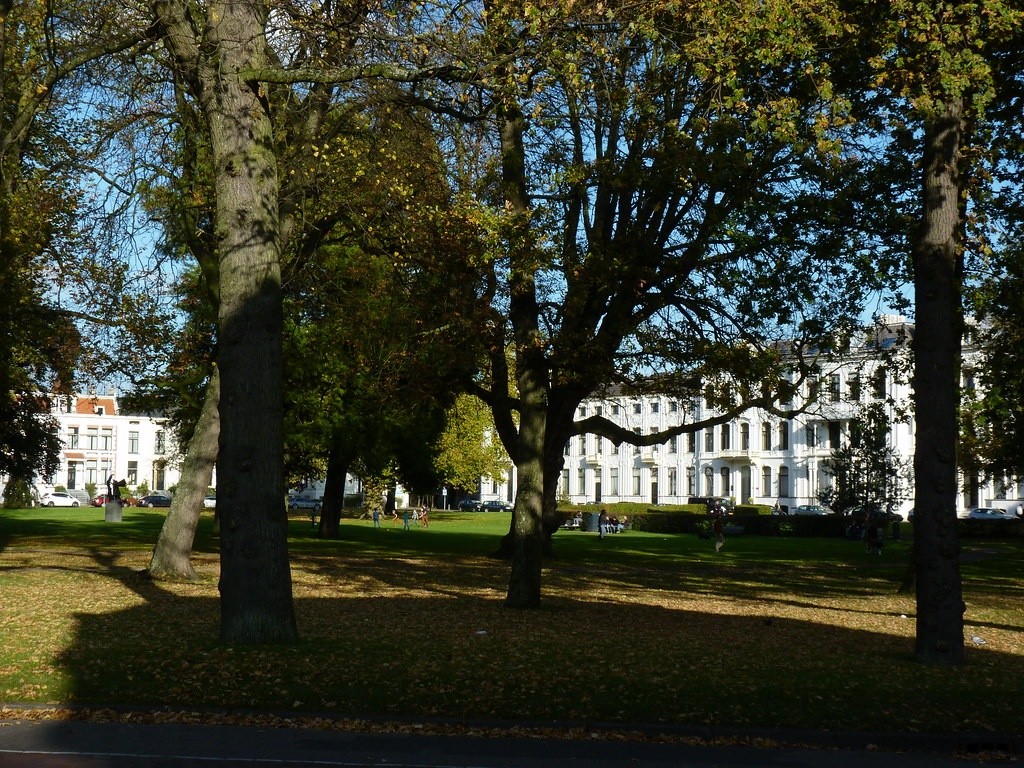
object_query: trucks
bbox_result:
[688,497,734,516]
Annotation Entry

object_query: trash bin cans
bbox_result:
[582,512,598,532]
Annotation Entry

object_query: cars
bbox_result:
[288,497,321,510]
[852,506,903,521]
[136,496,171,507]
[203,497,216,507]
[458,500,483,512]
[91,494,130,507]
[969,508,1019,519]
[907,508,914,521]
[795,505,828,515]
[40,492,81,508]
[480,500,511,512]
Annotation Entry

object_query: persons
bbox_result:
[403,511,409,531]
[908,509,914,522]
[851,505,903,551]
[775,500,781,511]
[710,499,725,552]
[598,509,628,540]
[392,510,403,528]
[312,509,316,527]
[571,511,582,526]
[373,508,380,528]
[412,505,429,526]
